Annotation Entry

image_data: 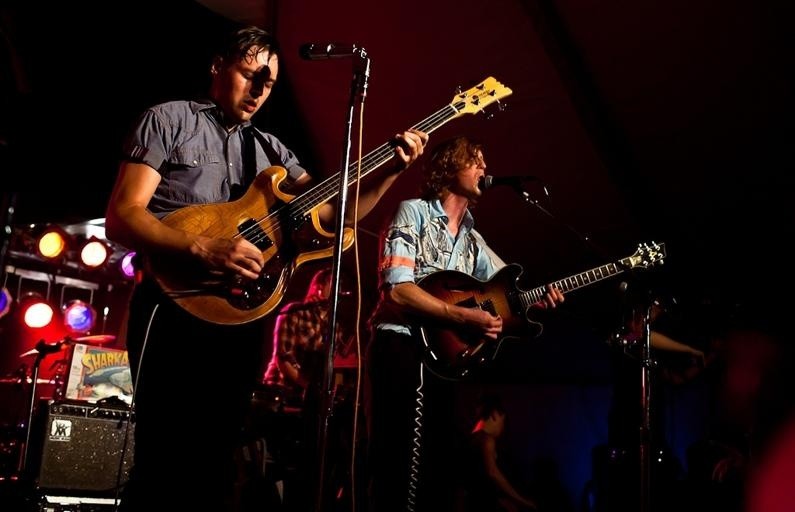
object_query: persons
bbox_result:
[106,24,429,512]
[362,135,565,512]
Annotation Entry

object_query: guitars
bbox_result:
[136,75,513,326]
[403,241,666,380]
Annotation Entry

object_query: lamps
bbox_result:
[29,225,65,262]
[76,234,108,271]
[0,284,11,321]
[117,250,142,279]
[64,299,97,332]
[18,292,53,329]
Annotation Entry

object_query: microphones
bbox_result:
[298,42,355,61]
[619,282,628,291]
[478,173,537,191]
